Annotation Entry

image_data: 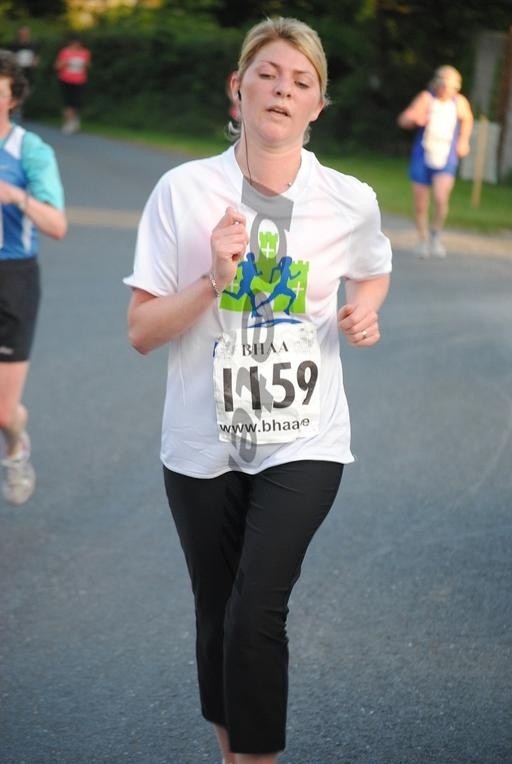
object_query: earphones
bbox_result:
[237,90,241,101]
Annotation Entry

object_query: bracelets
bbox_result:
[199,271,222,300]
[17,189,29,210]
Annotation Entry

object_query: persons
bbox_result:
[1,24,42,108]
[122,17,393,764]
[0,51,66,506]
[398,63,474,261]
[54,37,92,135]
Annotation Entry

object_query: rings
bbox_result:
[361,329,368,340]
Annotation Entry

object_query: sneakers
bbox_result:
[0,429,39,508]
[417,226,449,259]
